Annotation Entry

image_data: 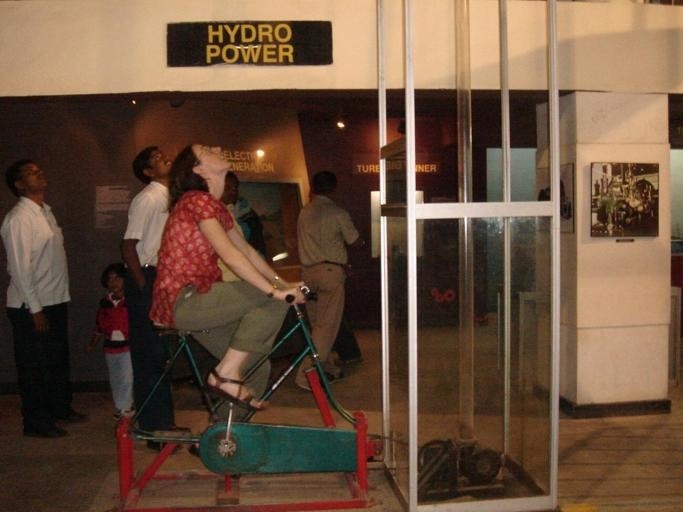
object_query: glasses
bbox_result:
[194,145,210,165]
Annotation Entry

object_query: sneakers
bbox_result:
[113,406,136,421]
[294,362,341,390]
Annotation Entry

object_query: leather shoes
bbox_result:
[24,427,66,437]
[59,414,86,421]
[146,427,192,449]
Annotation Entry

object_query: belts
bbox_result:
[325,261,343,267]
[142,264,155,274]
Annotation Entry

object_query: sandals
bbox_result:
[189,444,240,479]
[207,367,265,411]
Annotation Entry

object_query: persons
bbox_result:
[150,144,306,456]
[95,262,136,422]
[122,146,191,448]
[220,171,266,262]
[294,169,371,392]
[0,160,86,438]
